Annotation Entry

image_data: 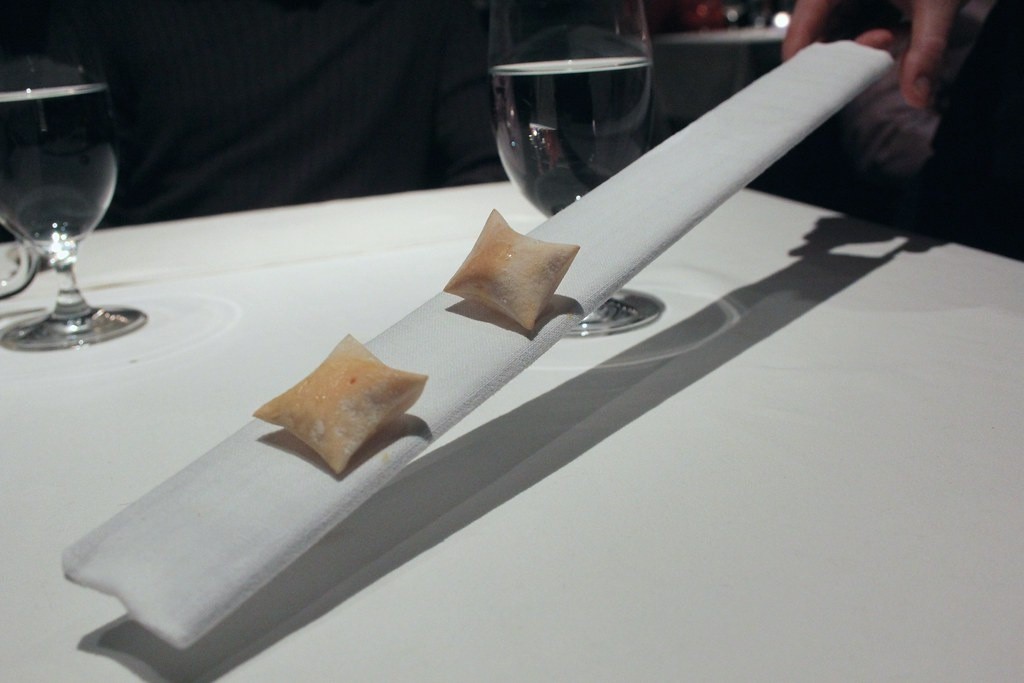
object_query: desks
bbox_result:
[0,169,1023,683]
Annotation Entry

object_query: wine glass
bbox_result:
[0,40,148,352]
[484,0,666,339]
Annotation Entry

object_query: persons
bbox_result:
[0,0,510,246]
[628,0,1024,258]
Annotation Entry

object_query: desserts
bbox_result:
[253,335,428,475]
[442,210,581,333]
